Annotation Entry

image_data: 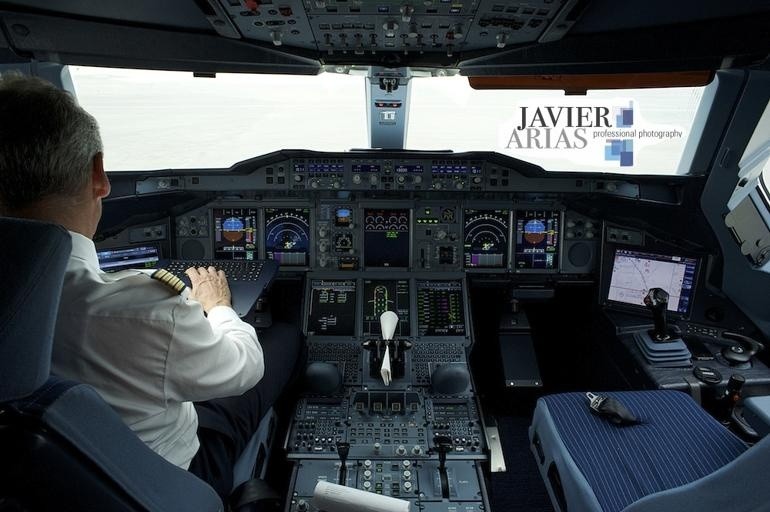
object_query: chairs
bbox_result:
[528,389,770,512]
[0,216,277,512]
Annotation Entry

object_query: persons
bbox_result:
[0,71,300,510]
